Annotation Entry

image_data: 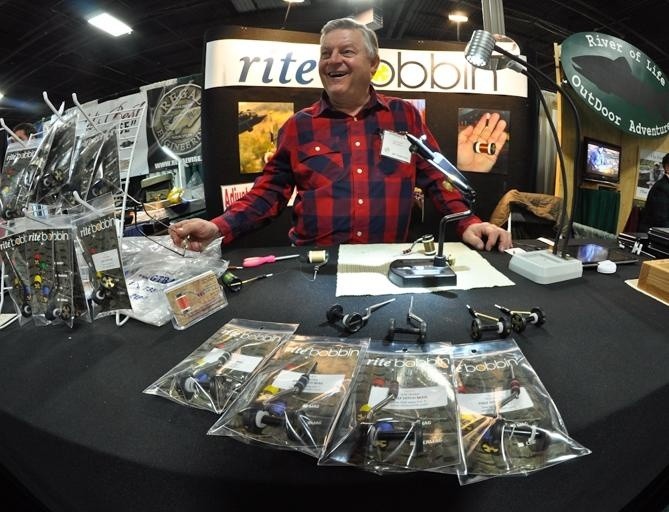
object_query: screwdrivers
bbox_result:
[243,254,304,267]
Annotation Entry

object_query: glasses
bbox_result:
[134,200,191,257]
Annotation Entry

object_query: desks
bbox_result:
[1,240,668,512]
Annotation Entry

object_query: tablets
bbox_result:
[555,243,638,267]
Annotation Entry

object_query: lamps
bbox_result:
[465,30,585,285]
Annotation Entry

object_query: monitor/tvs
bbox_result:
[581,136,622,187]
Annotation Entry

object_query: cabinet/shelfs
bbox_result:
[577,187,620,233]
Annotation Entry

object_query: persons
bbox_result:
[457,112,508,172]
[168,17,513,253]
[12,123,37,142]
[589,145,605,168]
[637,153,669,232]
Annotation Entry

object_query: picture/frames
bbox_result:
[634,144,669,201]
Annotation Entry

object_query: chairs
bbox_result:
[503,191,570,239]
